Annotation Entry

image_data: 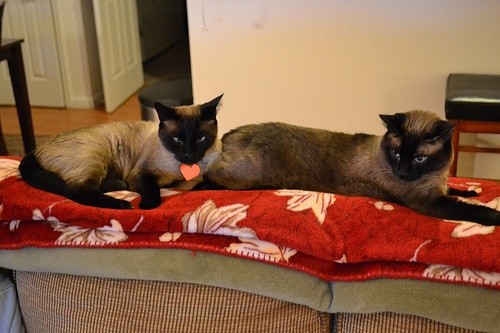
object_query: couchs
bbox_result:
[0,155,499,333]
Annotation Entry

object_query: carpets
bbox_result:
[4,134,51,156]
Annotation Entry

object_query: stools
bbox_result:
[444,73,500,182]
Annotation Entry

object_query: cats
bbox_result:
[18,93,238,210]
[203,109,500,230]
